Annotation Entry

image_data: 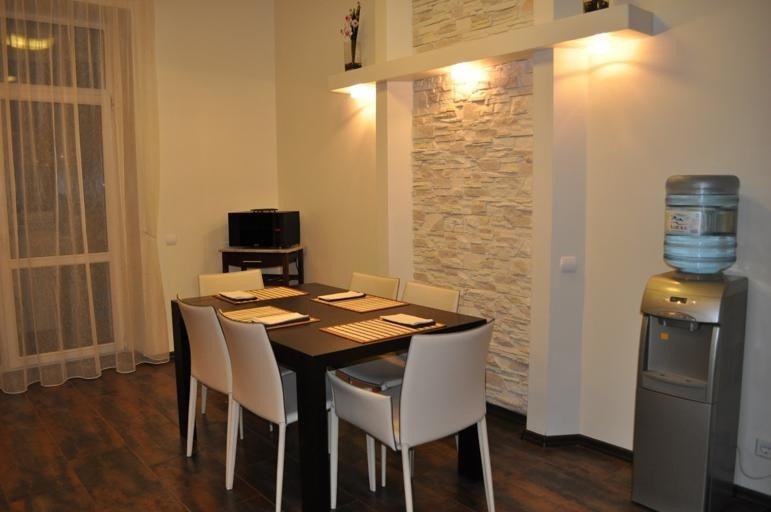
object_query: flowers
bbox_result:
[338,1,361,62]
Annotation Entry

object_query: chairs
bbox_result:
[197,269,267,441]
[328,282,461,493]
[349,273,400,299]
[217,308,333,512]
[177,296,273,490]
[326,321,493,512]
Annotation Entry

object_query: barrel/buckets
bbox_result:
[662,173,738,276]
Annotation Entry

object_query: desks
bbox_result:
[171,281,486,512]
[218,244,304,287]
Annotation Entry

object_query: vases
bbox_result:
[342,35,361,70]
